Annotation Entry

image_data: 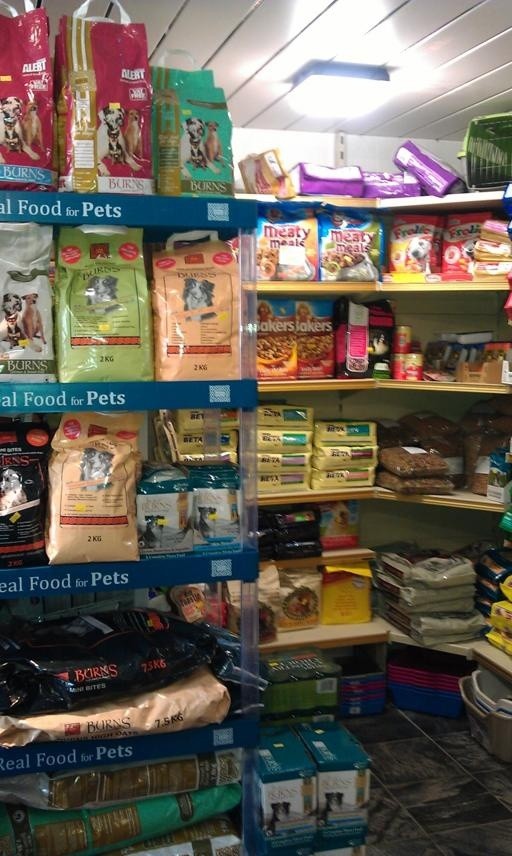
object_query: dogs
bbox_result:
[263,802,291,838]
[79,448,114,491]
[325,503,350,538]
[288,593,311,619]
[0,293,46,352]
[0,96,46,164]
[183,279,218,321]
[318,793,344,827]
[406,237,433,273]
[87,276,128,317]
[198,507,216,539]
[0,468,27,512]
[138,515,165,550]
[180,117,225,181]
[97,107,151,177]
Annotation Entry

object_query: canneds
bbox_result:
[390,324,424,380]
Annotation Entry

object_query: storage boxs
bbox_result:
[290,715,374,841]
[246,719,318,851]
[258,710,339,724]
[260,648,342,709]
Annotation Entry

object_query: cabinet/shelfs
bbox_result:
[234,187,512,692]
[0,175,270,851]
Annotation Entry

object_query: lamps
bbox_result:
[280,59,395,123]
